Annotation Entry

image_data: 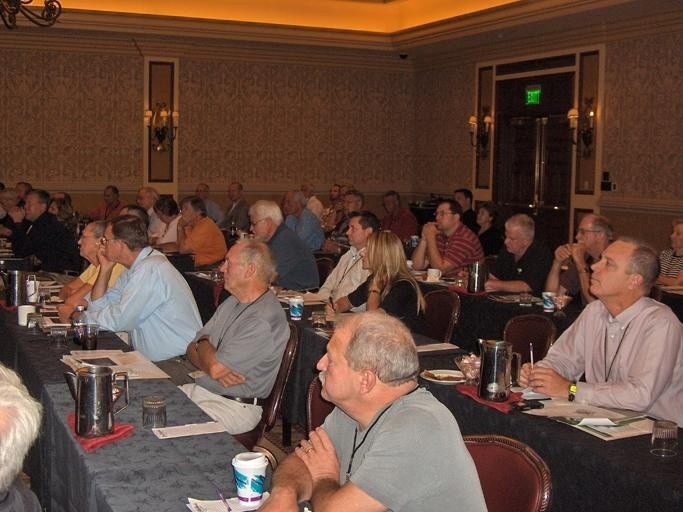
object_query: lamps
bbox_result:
[469,115,493,160]
[567,108,592,150]
[143,102,180,153]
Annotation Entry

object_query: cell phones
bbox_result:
[509,399,544,411]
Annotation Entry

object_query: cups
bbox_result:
[288,296,303,320]
[142,393,168,431]
[649,419,678,459]
[409,235,418,248]
[80,322,99,350]
[452,354,481,386]
[426,269,441,282]
[519,287,532,306]
[25,280,39,303]
[310,310,326,330]
[231,451,269,505]
[541,291,556,314]
[17,304,68,351]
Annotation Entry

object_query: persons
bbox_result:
[1,177,682,511]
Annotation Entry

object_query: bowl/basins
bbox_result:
[548,294,573,311]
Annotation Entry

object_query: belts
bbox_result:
[219,394,266,408]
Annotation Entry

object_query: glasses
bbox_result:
[248,217,267,226]
[101,236,122,245]
[433,210,457,217]
[575,228,602,235]
[24,200,44,208]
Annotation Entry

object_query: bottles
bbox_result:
[229,222,237,237]
[70,305,88,345]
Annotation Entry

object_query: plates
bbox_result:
[420,369,465,386]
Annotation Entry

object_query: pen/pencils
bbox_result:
[529,342,533,371]
[330,296,336,310]
[216,488,232,512]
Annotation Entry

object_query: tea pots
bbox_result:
[475,337,520,403]
[466,260,489,294]
[0,268,37,308]
[64,365,130,439]
